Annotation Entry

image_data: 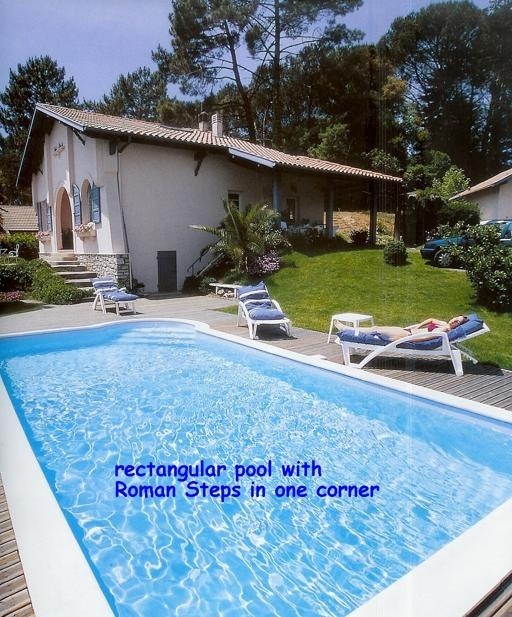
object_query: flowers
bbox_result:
[35,231,50,239]
[71,222,95,237]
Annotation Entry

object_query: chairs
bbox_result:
[0,243,19,265]
[235,281,292,341]
[90,275,138,316]
[335,312,491,376]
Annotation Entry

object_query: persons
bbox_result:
[334,314,471,343]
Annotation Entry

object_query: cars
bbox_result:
[419,216,512,269]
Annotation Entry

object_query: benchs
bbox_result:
[209,282,242,298]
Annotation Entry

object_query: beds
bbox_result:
[326,312,375,343]
